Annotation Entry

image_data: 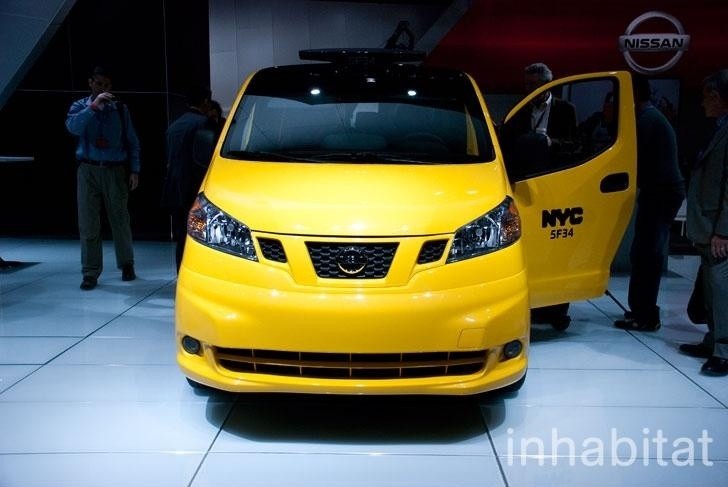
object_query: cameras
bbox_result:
[105,95,118,103]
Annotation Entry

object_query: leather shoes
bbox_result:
[678,343,728,376]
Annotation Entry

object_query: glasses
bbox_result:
[93,79,110,88]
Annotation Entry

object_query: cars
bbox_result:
[175,45,638,394]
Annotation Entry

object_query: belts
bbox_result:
[81,157,126,166]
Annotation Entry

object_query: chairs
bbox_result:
[277,103,451,156]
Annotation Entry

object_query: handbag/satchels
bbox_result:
[687,265,713,324]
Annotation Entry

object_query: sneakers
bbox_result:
[121,267,135,280]
[80,276,97,290]
[615,311,660,331]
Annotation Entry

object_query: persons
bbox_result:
[155,84,219,275]
[674,68,728,377]
[65,62,142,290]
[507,60,581,332]
[607,67,686,332]
[204,98,223,139]
